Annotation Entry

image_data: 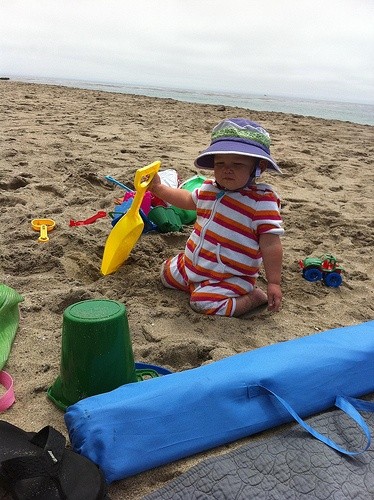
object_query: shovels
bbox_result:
[105,174,133,193]
[32,218,55,242]
[101,160,161,276]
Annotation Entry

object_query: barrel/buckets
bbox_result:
[147,205,183,233]
[48,300,173,411]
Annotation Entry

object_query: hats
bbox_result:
[193,118,283,177]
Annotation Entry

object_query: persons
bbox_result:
[141,118,284,317]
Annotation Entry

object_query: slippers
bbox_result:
[0,420,105,500]
